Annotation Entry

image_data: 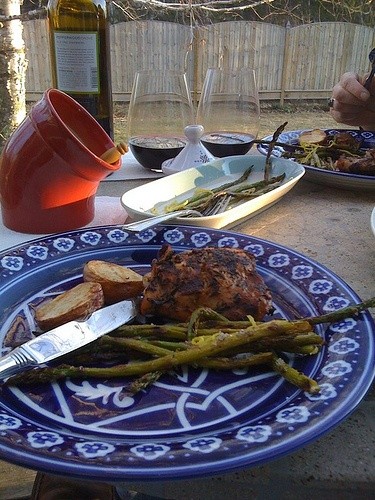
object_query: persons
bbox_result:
[327,71,375,131]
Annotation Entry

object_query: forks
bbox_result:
[123,193,232,232]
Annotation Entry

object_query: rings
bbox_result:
[327,98,336,107]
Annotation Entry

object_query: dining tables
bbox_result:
[1,136,375,500]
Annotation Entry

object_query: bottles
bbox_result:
[45,1,117,144]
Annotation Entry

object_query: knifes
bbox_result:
[1,297,138,382]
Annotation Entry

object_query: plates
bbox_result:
[257,128,375,191]
[0,223,375,479]
[120,155,307,230]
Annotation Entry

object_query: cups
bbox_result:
[126,70,195,172]
[195,68,261,159]
[0,88,123,235]
